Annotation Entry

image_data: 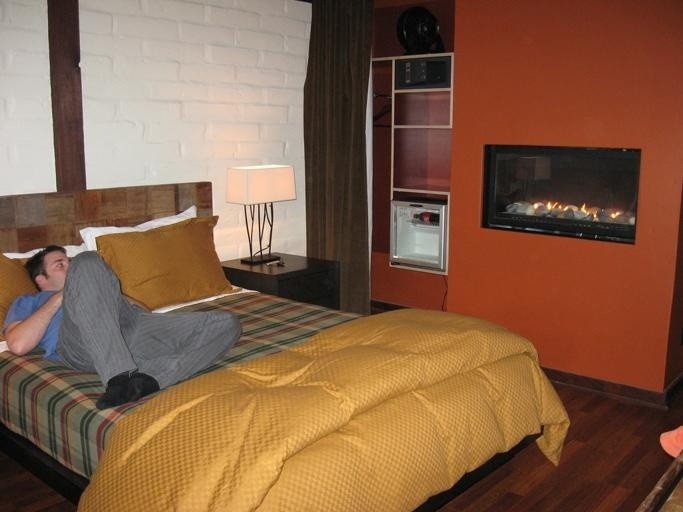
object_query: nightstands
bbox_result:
[221,251,342,311]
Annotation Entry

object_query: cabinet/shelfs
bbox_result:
[369,49,456,312]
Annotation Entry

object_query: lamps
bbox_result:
[221,163,298,267]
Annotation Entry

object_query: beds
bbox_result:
[0,179,570,512]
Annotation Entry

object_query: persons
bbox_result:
[2,245,243,411]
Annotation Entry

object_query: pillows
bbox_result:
[1,204,244,355]
